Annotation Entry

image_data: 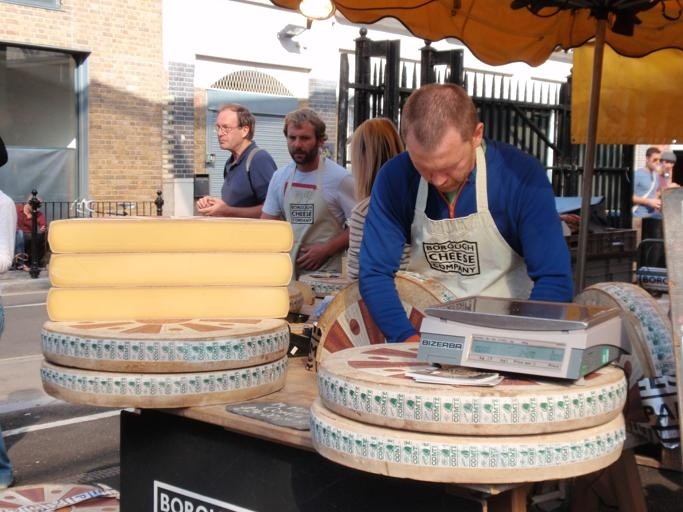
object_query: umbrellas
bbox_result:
[271,1,682,295]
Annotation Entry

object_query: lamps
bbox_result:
[275,0,336,40]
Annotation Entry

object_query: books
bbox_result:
[405,368,504,387]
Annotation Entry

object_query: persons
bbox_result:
[17,201,46,271]
[262,108,357,281]
[196,104,278,218]
[0,139,20,491]
[360,82,574,342]
[654,152,681,213]
[346,118,404,281]
[632,147,663,283]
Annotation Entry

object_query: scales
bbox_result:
[417,294,621,385]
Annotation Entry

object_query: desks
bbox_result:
[119,355,652,512]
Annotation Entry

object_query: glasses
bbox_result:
[653,159,663,162]
[214,125,239,133]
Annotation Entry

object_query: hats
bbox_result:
[664,152,677,161]
